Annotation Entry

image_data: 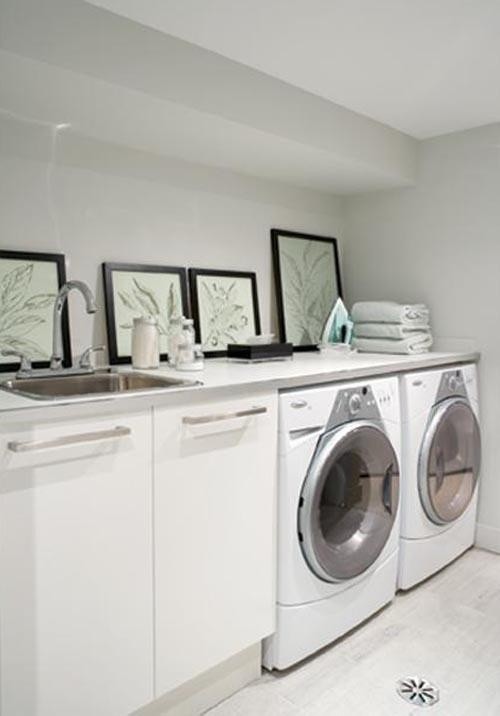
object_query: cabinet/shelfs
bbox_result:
[0,381,276,716]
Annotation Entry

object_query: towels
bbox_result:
[350,302,432,353]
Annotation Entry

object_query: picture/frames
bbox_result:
[101,261,190,366]
[0,248,74,372]
[187,267,262,359]
[270,228,346,354]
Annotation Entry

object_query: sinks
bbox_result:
[1,366,203,403]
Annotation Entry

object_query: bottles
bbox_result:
[167,314,205,372]
[131,317,160,369]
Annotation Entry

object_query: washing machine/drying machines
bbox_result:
[263,375,403,672]
[398,363,483,593]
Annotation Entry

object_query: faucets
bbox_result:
[48,279,98,372]
